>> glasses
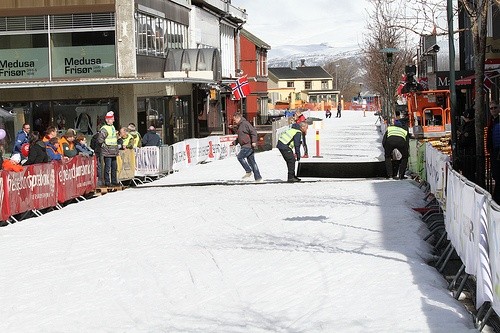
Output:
[489,106,498,109]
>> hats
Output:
[63,111,155,137]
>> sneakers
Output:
[392,174,407,180]
[243,172,262,182]
[288,176,301,182]
[105,182,120,187]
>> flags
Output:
[483,72,493,93]
[231,75,251,100]
[398,74,410,98]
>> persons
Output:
[325,109,332,118]
[292,110,310,159]
[336,101,342,117]
[230,112,263,183]
[455,108,475,183]
[91,125,104,184]
[96,110,122,188]
[488,99,500,206]
[284,108,290,117]
[382,120,410,181]
[0,123,95,170]
[276,121,309,182]
[394,111,402,127]
[115,122,160,150]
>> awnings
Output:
[453,71,491,85]
